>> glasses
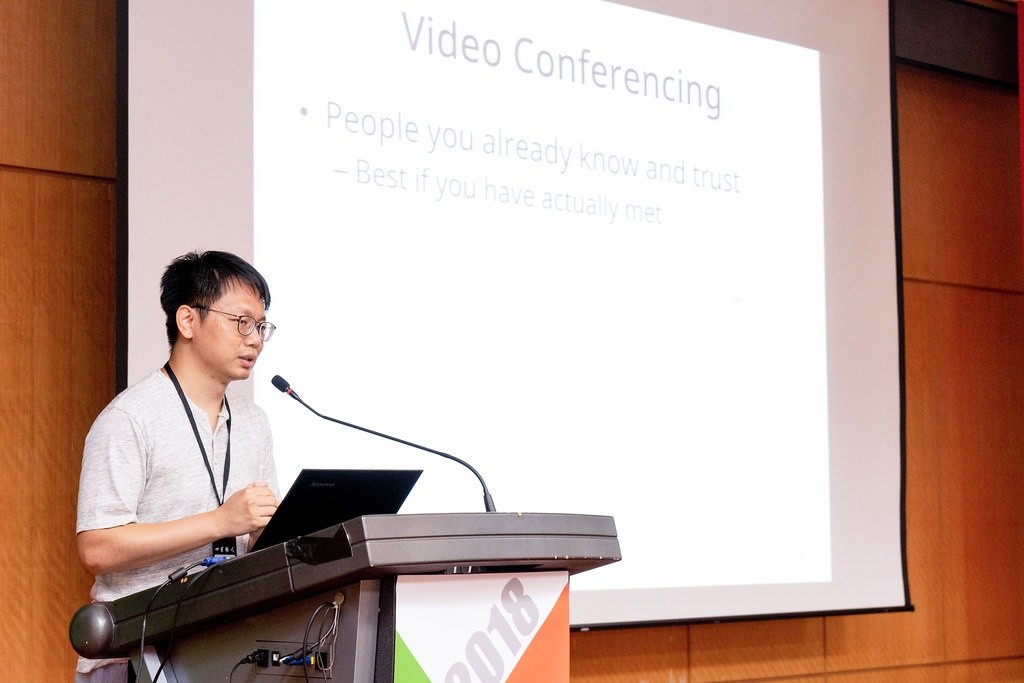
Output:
[192,305,276,342]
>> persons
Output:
[74,251,281,683]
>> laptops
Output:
[251,469,424,551]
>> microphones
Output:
[269,375,495,512]
